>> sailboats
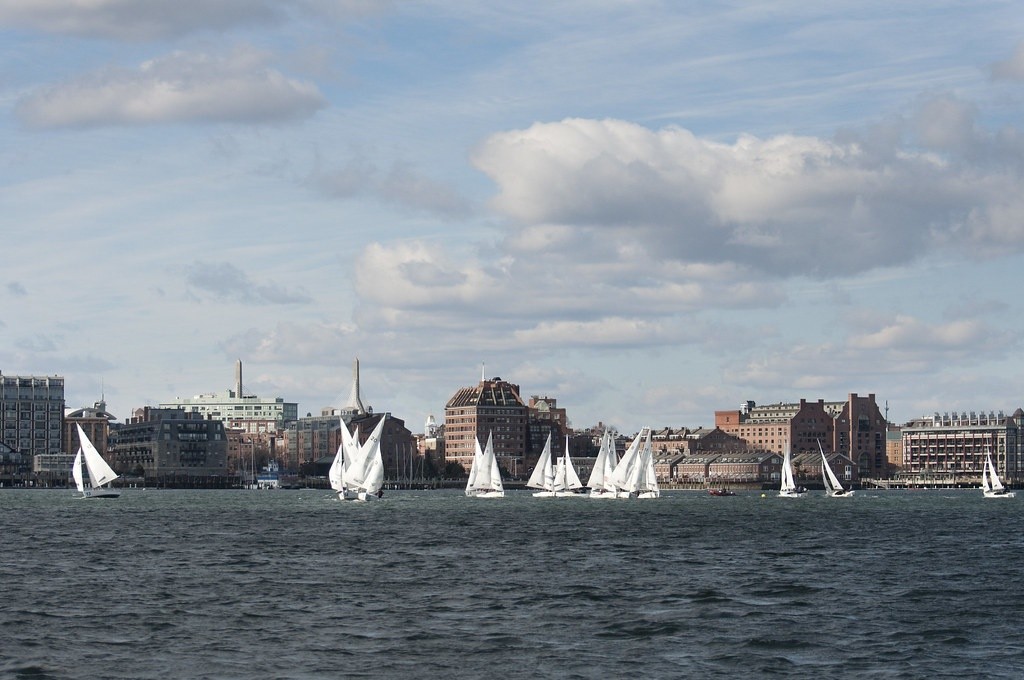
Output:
[816,438,855,498]
[464,428,505,497]
[585,427,663,499]
[525,429,592,499]
[72,421,122,498]
[328,411,386,501]
[776,442,808,498]
[982,437,1016,498]
